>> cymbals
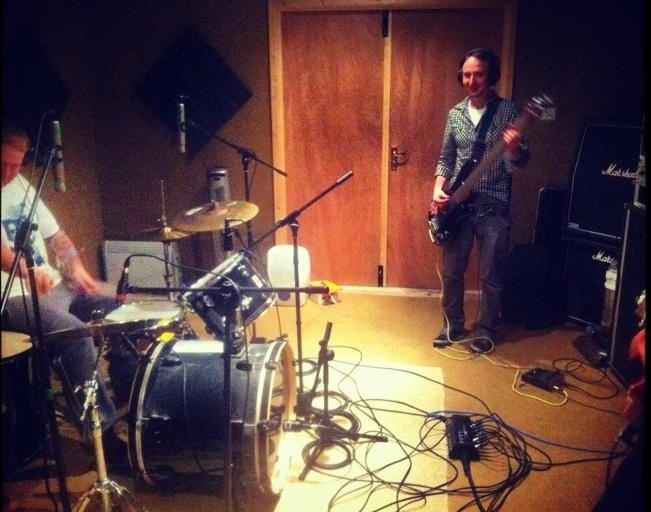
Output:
[23,316,162,343]
[170,200,259,232]
[148,226,197,240]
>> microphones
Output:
[179,102,187,155]
[116,258,130,302]
[224,220,233,258]
[50,120,66,193]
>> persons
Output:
[1,123,132,478]
[431,44,531,354]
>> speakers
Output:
[534,189,650,333]
[567,123,651,239]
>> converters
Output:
[521,372,561,392]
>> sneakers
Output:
[432,326,464,344]
[471,336,495,352]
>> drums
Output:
[126,337,297,496]
[105,301,199,361]
[183,252,278,340]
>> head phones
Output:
[457,49,500,86]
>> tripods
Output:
[71,333,148,512]
[298,320,388,480]
[248,171,361,428]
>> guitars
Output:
[427,92,555,247]
